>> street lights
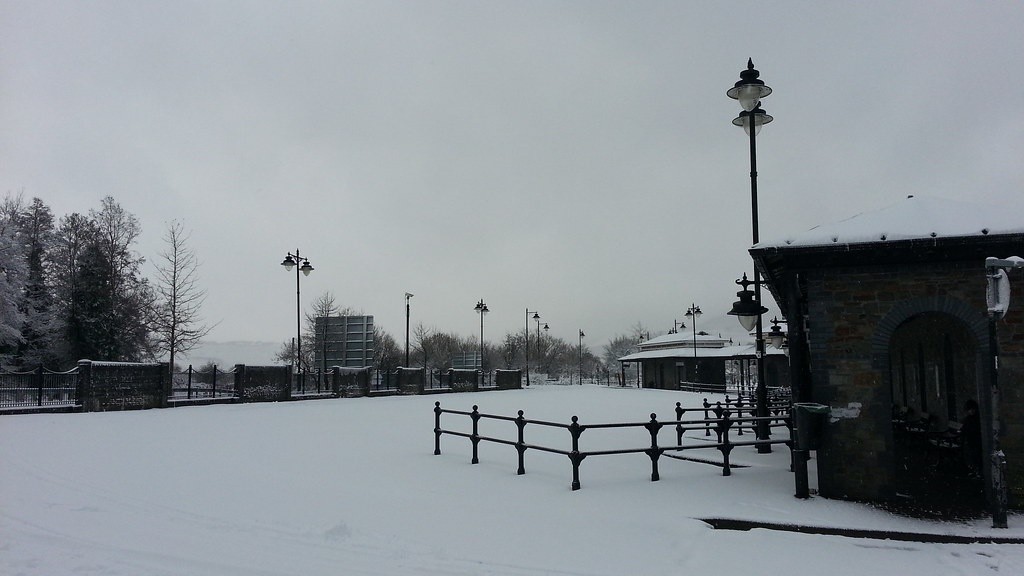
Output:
[473,298,491,387]
[537,321,550,353]
[282,249,315,391]
[674,319,686,334]
[640,331,650,342]
[684,302,704,384]
[525,307,541,387]
[726,55,776,455]
[578,329,586,386]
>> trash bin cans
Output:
[793,402,830,458]
